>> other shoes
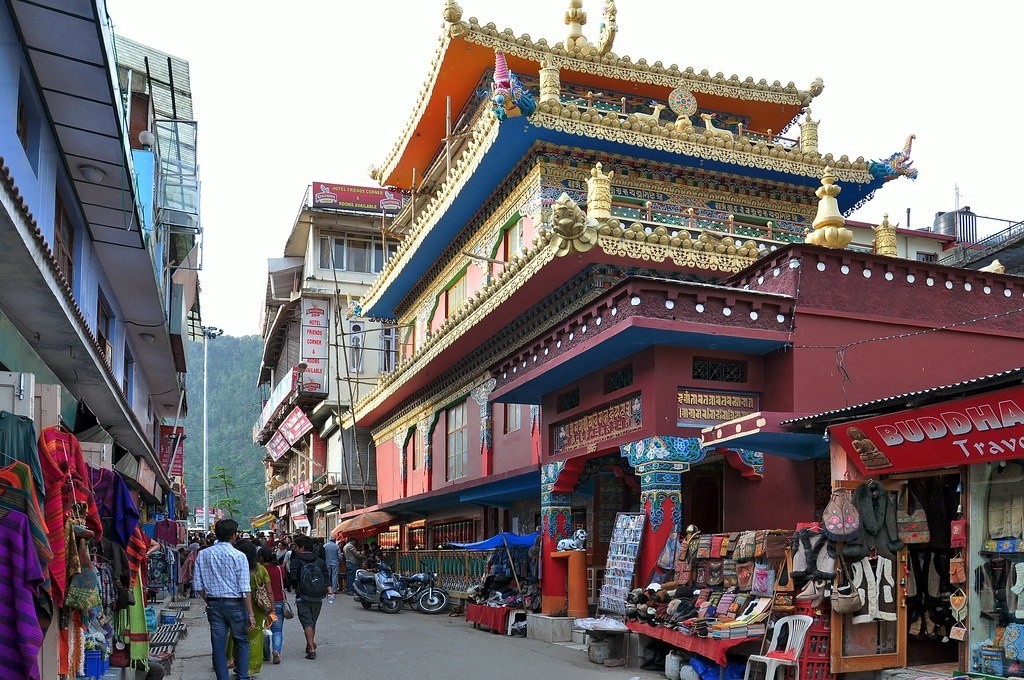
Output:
[306,642,316,653]
[273,651,281,664]
[263,656,271,661]
[306,652,316,659]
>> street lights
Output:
[198,326,225,535]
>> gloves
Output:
[655,603,668,619]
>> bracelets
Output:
[248,614,255,617]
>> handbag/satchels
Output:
[255,563,271,614]
[830,552,862,614]
[283,601,294,619]
[950,588,968,620]
[950,620,967,641]
[949,552,966,584]
[896,484,930,544]
[676,530,795,611]
[951,516,966,548]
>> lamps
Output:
[139,129,155,151]
[162,404,174,410]
[79,165,107,184]
[139,334,156,344]
[276,475,289,483]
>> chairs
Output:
[744,615,814,680]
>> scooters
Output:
[352,558,404,614]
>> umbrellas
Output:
[331,510,399,549]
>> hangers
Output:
[849,546,893,570]
[156,512,176,526]
[0,415,121,565]
[972,553,1019,576]
[149,539,177,554]
[797,522,831,536]
[852,479,892,492]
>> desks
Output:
[466,603,535,636]
[622,621,764,680]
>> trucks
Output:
[194,506,224,531]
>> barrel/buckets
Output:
[160,609,178,625]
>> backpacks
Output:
[298,559,325,600]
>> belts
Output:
[206,597,241,602]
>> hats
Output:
[674,586,695,600]
[242,533,251,538]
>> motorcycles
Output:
[391,560,450,615]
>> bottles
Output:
[327,596,334,603]
[973,658,979,672]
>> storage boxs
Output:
[784,600,837,680]
[75,641,110,680]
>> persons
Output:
[179,531,383,599]
[224,539,288,680]
[290,536,333,660]
[192,519,256,680]
